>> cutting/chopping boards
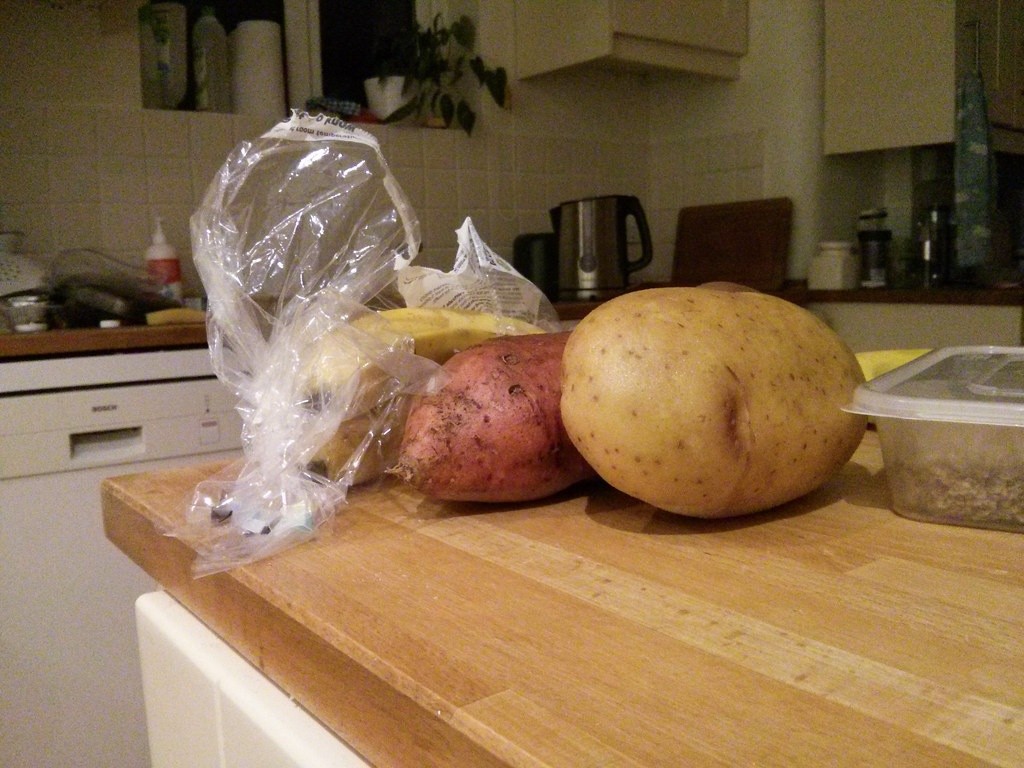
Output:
[670,196,792,298]
[99,428,1024,768]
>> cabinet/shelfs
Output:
[512,0,749,81]
[820,0,1024,156]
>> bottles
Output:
[192,6,231,111]
[805,240,860,291]
[144,219,184,307]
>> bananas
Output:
[296,306,552,488]
[849,347,935,382]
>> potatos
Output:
[560,285,869,519]
[390,332,619,504]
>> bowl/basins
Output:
[842,342,1024,535]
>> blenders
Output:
[856,206,892,289]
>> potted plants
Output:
[361,17,509,136]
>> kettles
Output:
[548,193,654,302]
